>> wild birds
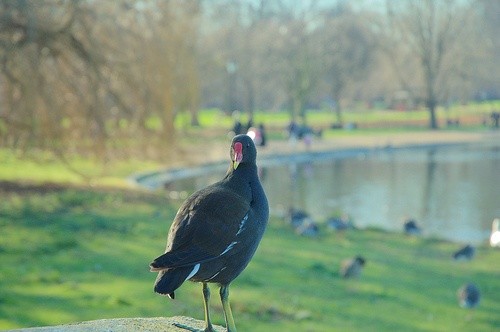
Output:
[458,284,479,306]
[403,221,420,235]
[489,218,500,248]
[287,208,308,224]
[149,134,269,332]
[452,245,475,261]
[338,256,365,278]
[295,223,319,237]
[325,216,347,232]
